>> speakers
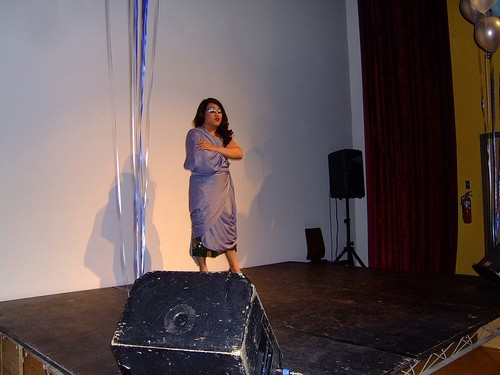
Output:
[110,270,284,375]
[472,241,500,284]
[328,148,365,200]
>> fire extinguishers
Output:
[460,190,474,224]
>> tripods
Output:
[335,199,367,268]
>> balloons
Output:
[459,0,500,53]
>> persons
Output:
[183,97,246,278]
